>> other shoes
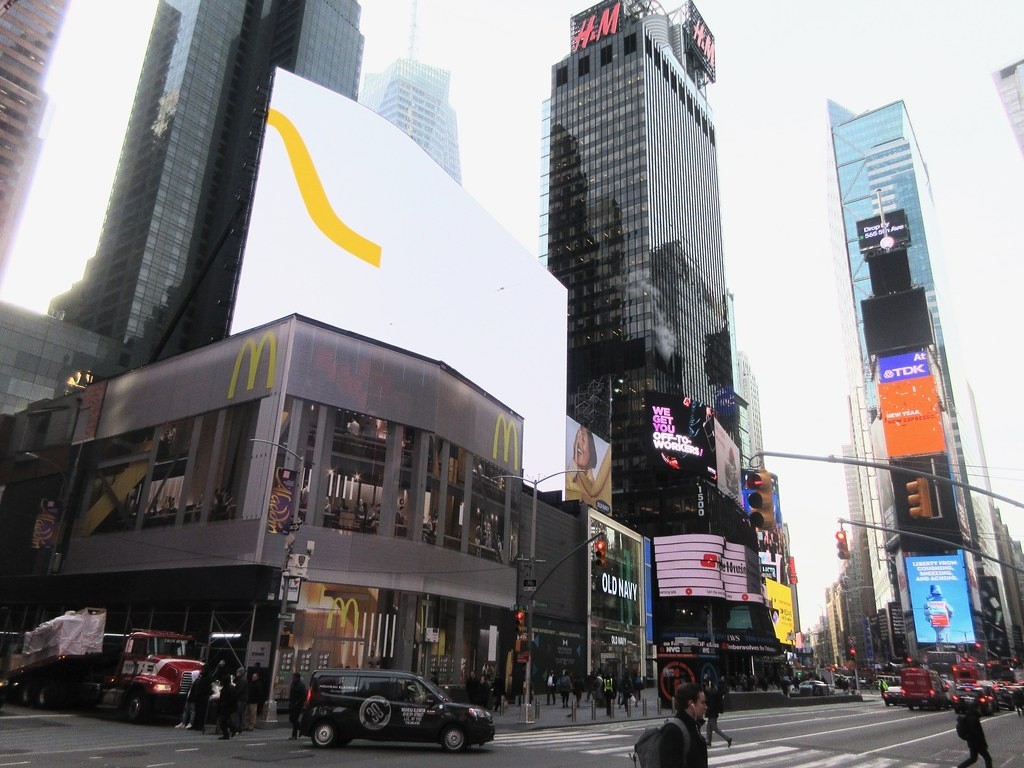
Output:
[175,722,186,729]
[219,736,232,740]
[727,738,732,747]
[186,724,192,729]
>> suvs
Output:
[302,667,497,753]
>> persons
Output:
[1012,687,1024,717]
[402,434,414,468]
[703,680,733,747]
[660,682,708,768]
[586,668,617,716]
[466,668,505,713]
[618,670,645,709]
[349,414,359,436]
[129,422,205,514]
[573,672,585,707]
[175,659,264,740]
[956,698,993,768]
[566,425,596,505]
[325,497,517,561]
[559,671,573,708]
[880,679,888,698]
[509,662,536,706]
[837,675,857,694]
[289,672,308,739]
[729,671,770,691]
[546,668,556,705]
[923,585,953,643]
[475,460,504,488]
[775,671,828,695]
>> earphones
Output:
[692,703,695,710]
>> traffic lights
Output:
[905,476,934,520]
[904,653,912,664]
[851,649,856,654]
[836,530,851,559]
[748,471,776,531]
[832,667,836,672]
[596,541,607,567]
[515,611,524,633]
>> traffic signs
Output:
[286,522,303,533]
[276,613,295,622]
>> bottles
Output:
[928,584,950,628]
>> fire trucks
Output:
[0,630,223,723]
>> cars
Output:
[861,663,1024,718]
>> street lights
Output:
[250,436,307,725]
[490,469,588,723]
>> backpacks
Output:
[632,717,690,768]
[955,715,968,739]
